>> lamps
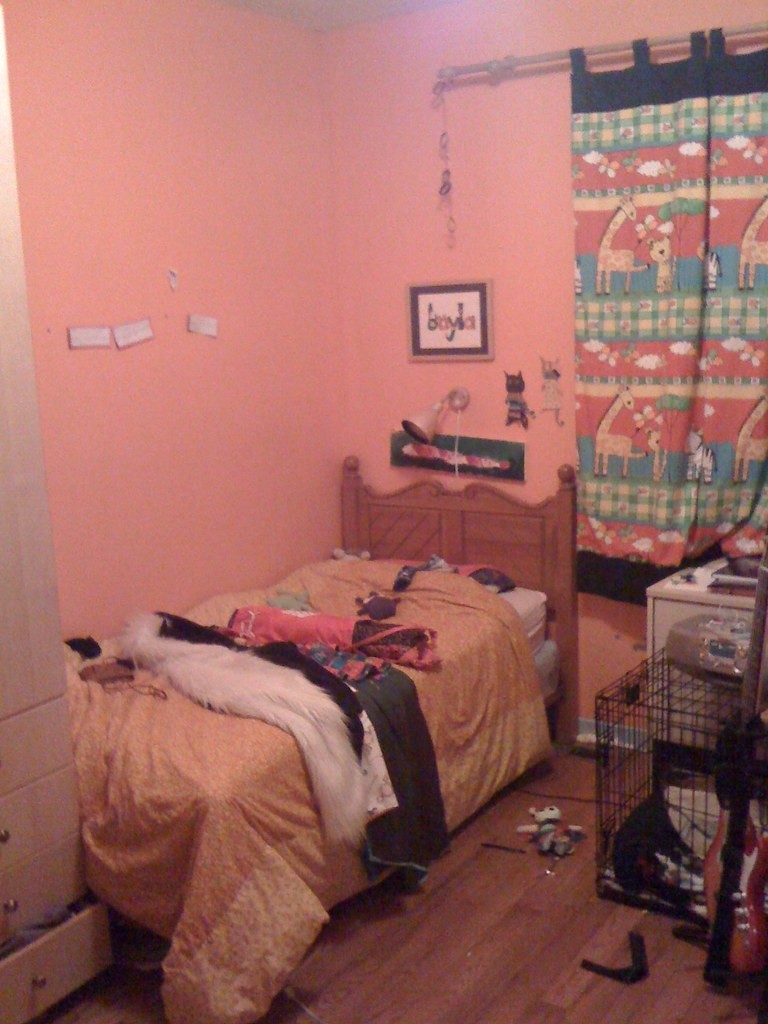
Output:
[404,384,471,444]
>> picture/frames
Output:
[409,280,497,363]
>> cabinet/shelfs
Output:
[643,564,766,755]
[1,0,115,1024]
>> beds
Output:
[61,452,579,1024]
